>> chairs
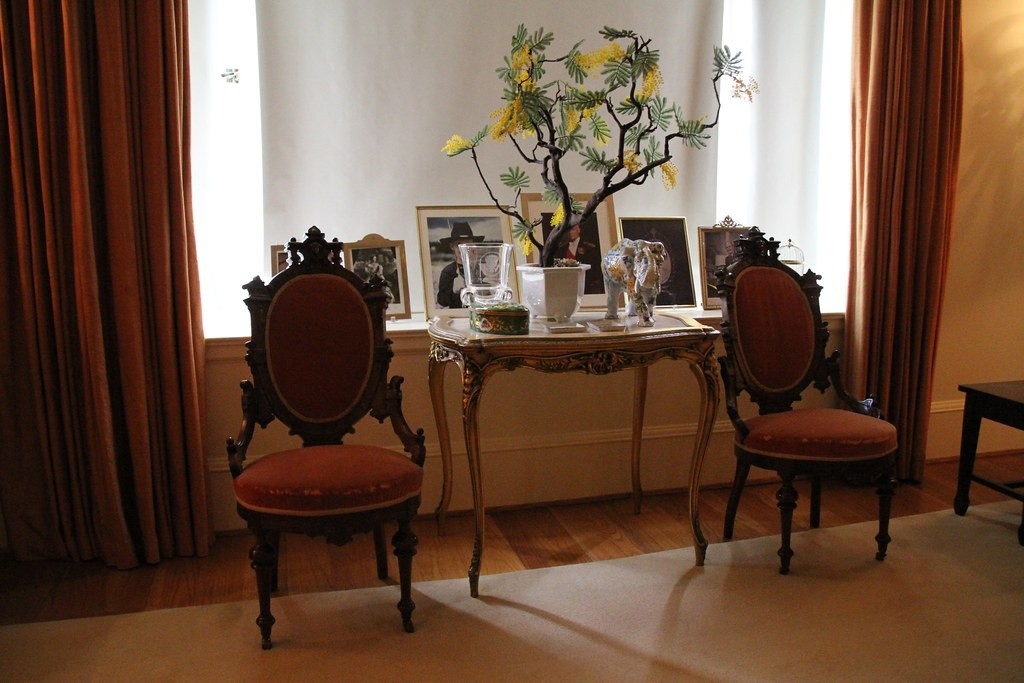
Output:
[714,225,901,573]
[226,226,429,649]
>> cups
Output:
[458,244,513,305]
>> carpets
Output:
[0,495,1024,683]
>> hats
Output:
[440,222,485,244]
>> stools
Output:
[951,376,1024,547]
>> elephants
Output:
[601,238,666,327]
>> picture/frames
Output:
[343,233,412,321]
[520,191,625,311]
[697,215,751,310]
[270,244,289,278]
[618,215,698,309]
[415,204,522,321]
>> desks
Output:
[425,307,722,596]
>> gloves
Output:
[453,278,465,294]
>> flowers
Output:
[438,15,761,265]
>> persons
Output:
[558,223,595,293]
[437,221,490,309]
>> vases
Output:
[514,258,593,318]
